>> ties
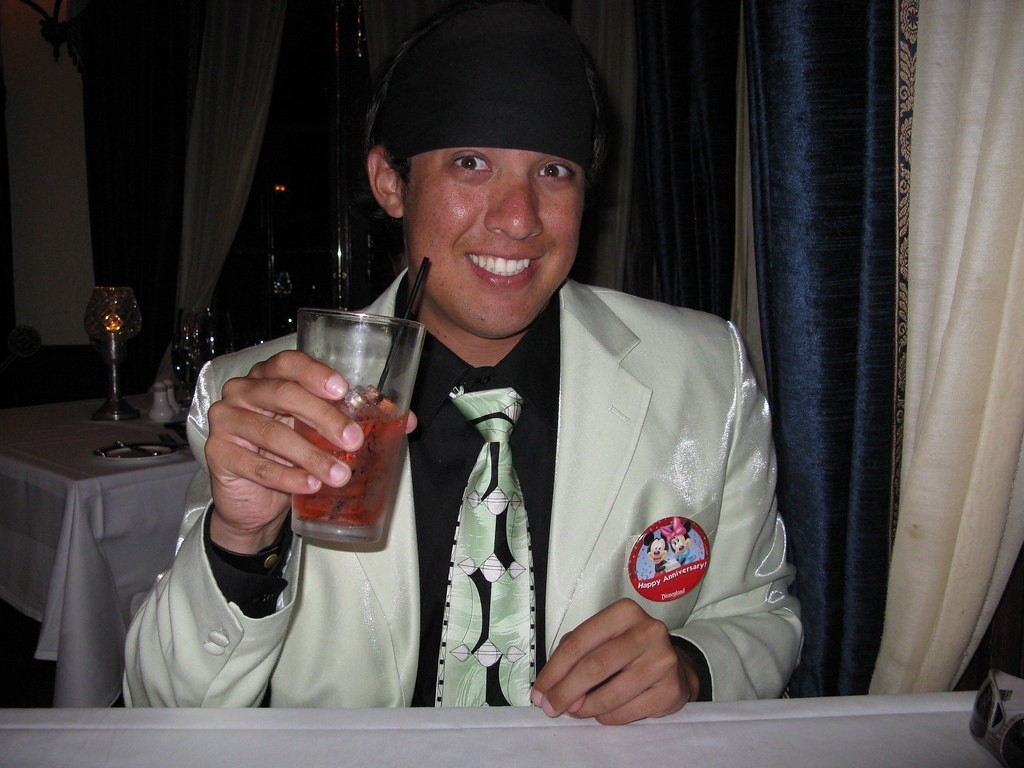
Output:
[432,385,536,706]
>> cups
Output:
[290,308,427,553]
[171,307,235,410]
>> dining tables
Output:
[0,394,201,708]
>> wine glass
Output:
[83,286,143,422]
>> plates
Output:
[93,439,184,460]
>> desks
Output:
[0,692,1023,768]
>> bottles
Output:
[148,377,180,423]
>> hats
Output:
[375,5,599,170]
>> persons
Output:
[120,1,805,726]
